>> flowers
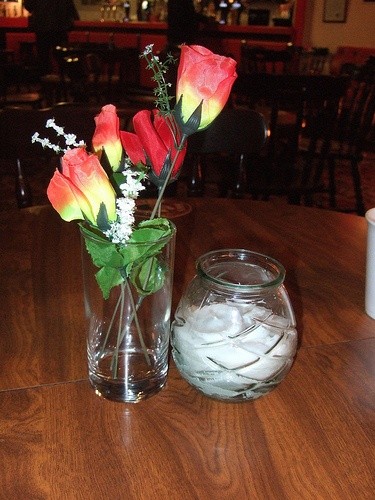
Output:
[32,44,237,379]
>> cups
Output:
[365,207,374,321]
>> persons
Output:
[165,0,221,110]
[193,0,217,22]
[22,0,80,79]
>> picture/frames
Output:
[322,0,348,23]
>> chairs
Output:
[186,45,375,217]
[0,42,139,174]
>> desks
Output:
[0,197,375,500]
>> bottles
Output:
[170,248,298,402]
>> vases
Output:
[78,215,177,405]
